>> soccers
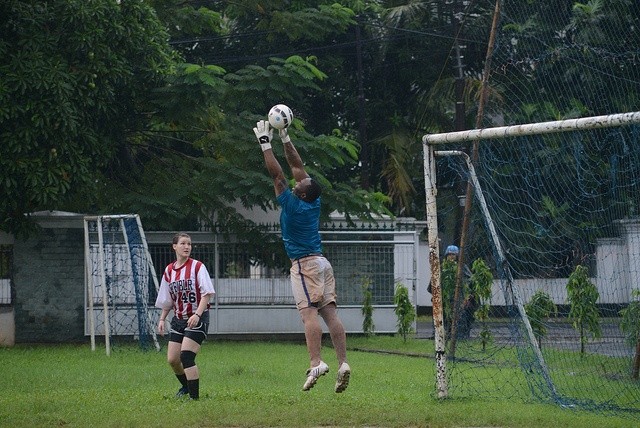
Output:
[268,103,294,130]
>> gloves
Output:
[252,119,274,153]
[279,126,291,145]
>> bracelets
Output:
[159,318,166,322]
[193,312,202,319]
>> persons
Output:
[154,231,217,403]
[252,119,352,394]
[427,244,482,342]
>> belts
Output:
[294,252,323,261]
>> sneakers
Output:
[334,362,351,394]
[303,361,329,391]
